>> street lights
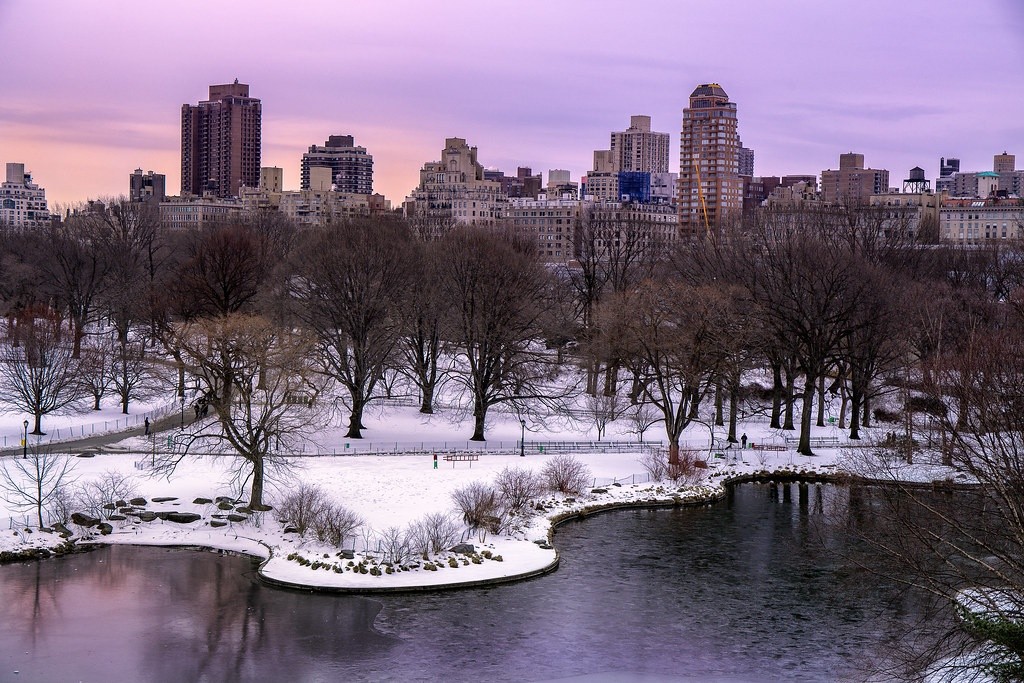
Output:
[23,420,30,458]
[711,413,715,445]
[275,415,281,450]
[520,420,526,456]
[928,415,933,448]
[179,398,185,431]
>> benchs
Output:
[787,437,838,445]
[522,440,664,450]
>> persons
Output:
[741,433,747,449]
[887,431,896,443]
[145,417,150,435]
[194,402,208,420]
